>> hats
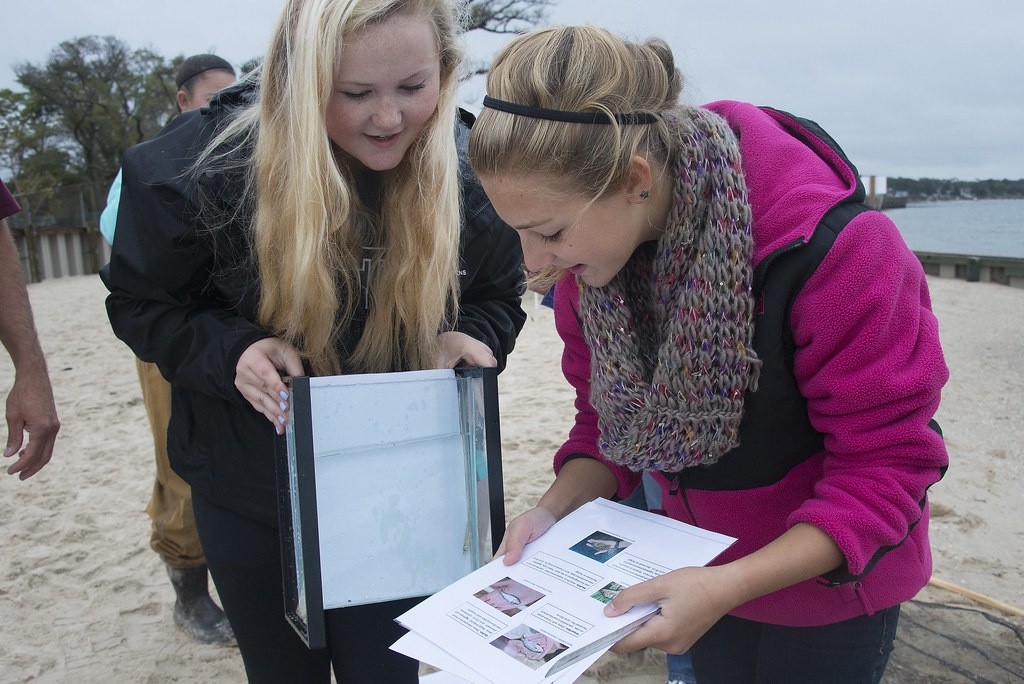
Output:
[177,55,235,89]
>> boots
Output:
[165,564,238,647]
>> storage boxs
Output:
[276,365,508,652]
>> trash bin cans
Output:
[966,257,981,281]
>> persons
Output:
[96,54,241,648]
[466,21,950,682]
[107,1,526,684]
[0,178,61,482]
